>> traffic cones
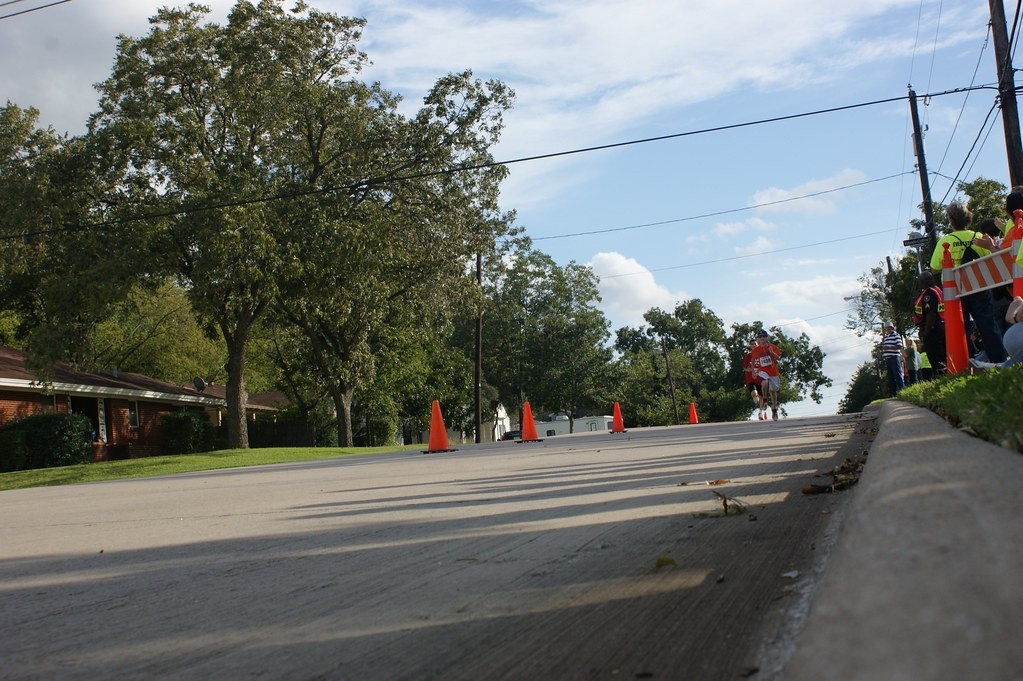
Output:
[609,402,628,434]
[687,403,701,424]
[515,400,544,443]
[421,400,460,454]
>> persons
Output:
[901,338,916,386]
[901,334,932,382]
[899,348,910,389]
[876,322,904,397]
[924,184,1023,377]
[744,338,766,419]
[750,330,781,420]
[914,272,946,380]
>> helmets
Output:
[918,271,933,288]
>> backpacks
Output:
[950,231,980,266]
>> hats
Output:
[883,322,894,330]
[756,330,768,337]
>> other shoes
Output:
[761,398,767,410]
[772,408,778,421]
[753,397,759,404]
[758,413,763,420]
